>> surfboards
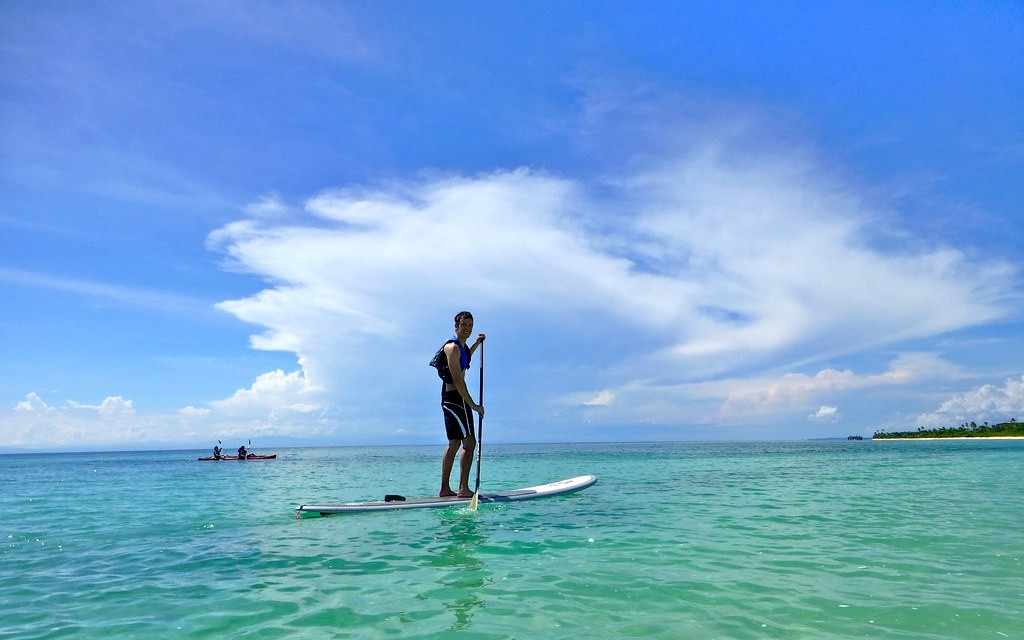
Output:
[294,474,599,512]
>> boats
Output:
[198,455,276,461]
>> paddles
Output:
[217,439,224,461]
[470,341,484,511]
[245,438,251,460]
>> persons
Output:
[214,446,222,457]
[440,311,486,497]
[238,446,247,458]
